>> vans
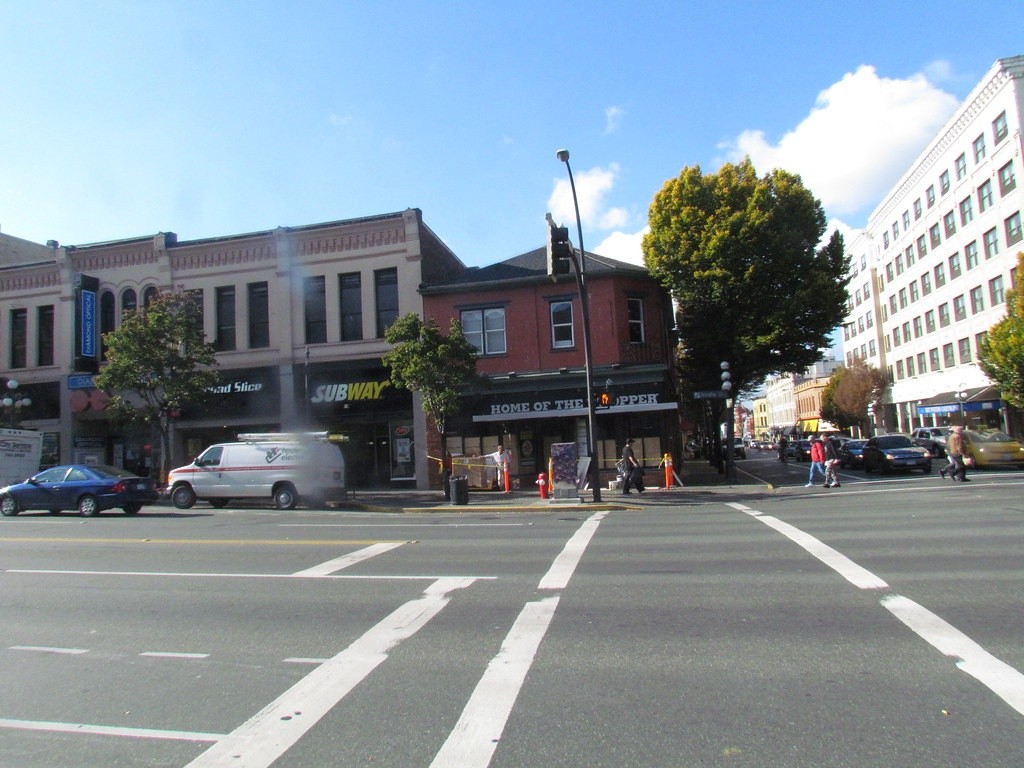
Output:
[167,432,348,511]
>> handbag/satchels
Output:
[961,454,972,466]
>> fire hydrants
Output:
[535,471,548,499]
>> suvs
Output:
[911,426,953,459]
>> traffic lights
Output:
[546,223,570,283]
[599,391,609,406]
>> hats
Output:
[626,439,636,443]
[806,435,814,442]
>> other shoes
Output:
[831,482,834,485]
[623,492,632,494]
[805,484,815,487]
[947,472,956,481]
[939,470,944,480]
[823,484,829,488]
[640,489,647,494]
[832,483,839,487]
[960,478,971,482]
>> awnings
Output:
[784,425,796,435]
[918,384,1003,414]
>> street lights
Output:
[0,378,33,430]
[954,391,968,430]
[556,145,602,502]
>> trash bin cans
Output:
[450,473,469,505]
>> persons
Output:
[778,437,788,460]
[949,426,972,482]
[936,429,955,479]
[820,433,841,488]
[804,435,825,487]
[622,439,646,494]
[481,446,511,490]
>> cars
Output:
[0,462,158,518]
[861,434,933,475]
[839,438,870,470]
[719,433,851,463]
[958,425,1024,470]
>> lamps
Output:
[970,363,976,366]
[611,364,620,369]
[911,376,918,379]
[345,400,349,410]
[559,368,569,374]
[938,370,943,374]
[508,372,518,378]
